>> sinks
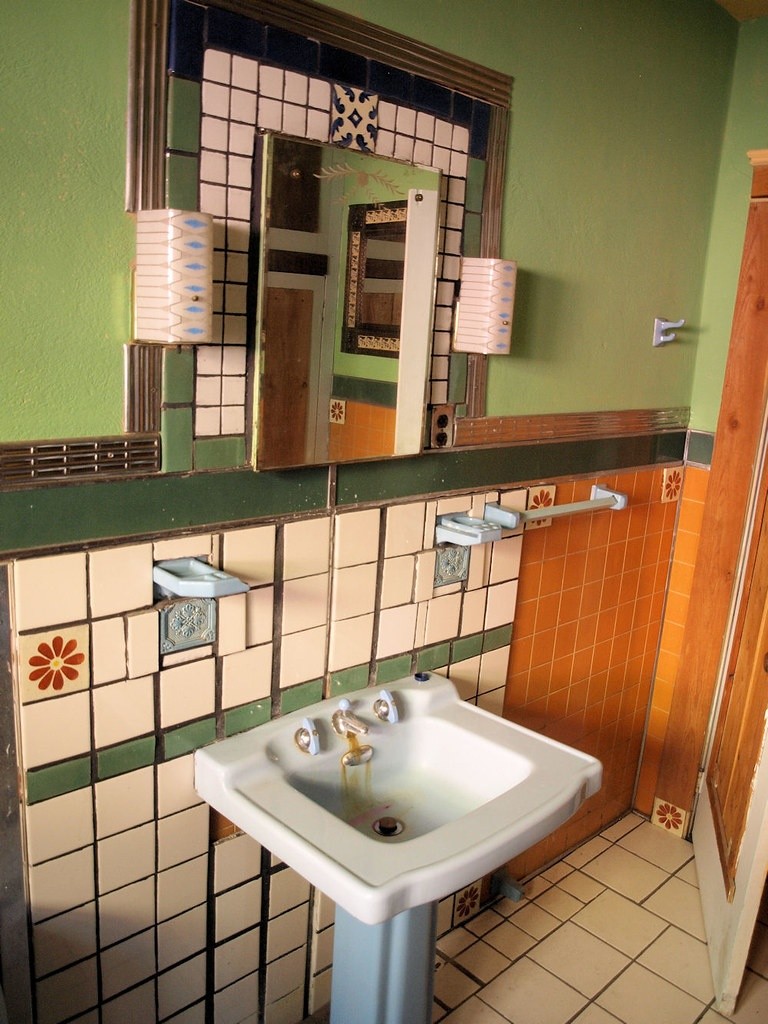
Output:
[193,669,603,926]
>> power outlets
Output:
[428,403,454,449]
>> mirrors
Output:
[122,0,516,436]
[340,199,408,359]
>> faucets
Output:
[331,697,370,736]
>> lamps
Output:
[134,208,213,355]
[452,256,517,356]
